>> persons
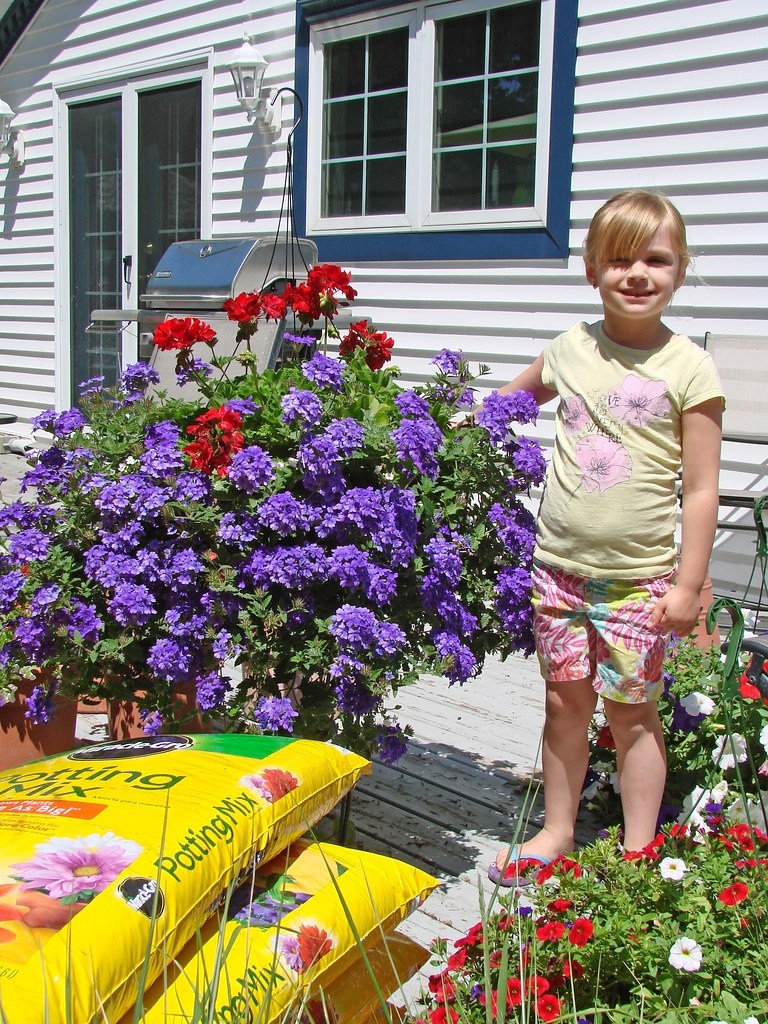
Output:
[472,189,726,887]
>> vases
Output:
[102,658,220,740]
[0,662,85,773]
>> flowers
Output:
[393,620,767,1023]
[0,263,550,764]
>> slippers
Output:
[489,843,555,887]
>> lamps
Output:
[225,27,283,135]
